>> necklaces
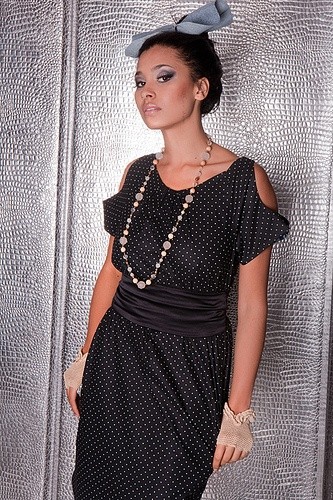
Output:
[118,132,214,289]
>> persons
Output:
[60,1,293,500]
